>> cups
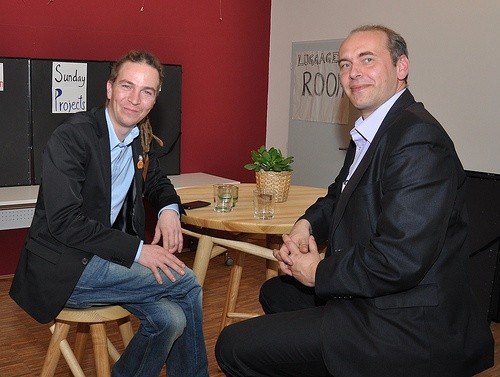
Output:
[252,189,275,220]
[213,184,239,214]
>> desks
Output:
[0,172,240,276]
[176,184,328,333]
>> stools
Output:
[40,306,133,377]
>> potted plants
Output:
[244,146,294,202]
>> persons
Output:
[214,22,496,377]
[8,50,208,377]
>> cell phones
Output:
[181,201,211,210]
